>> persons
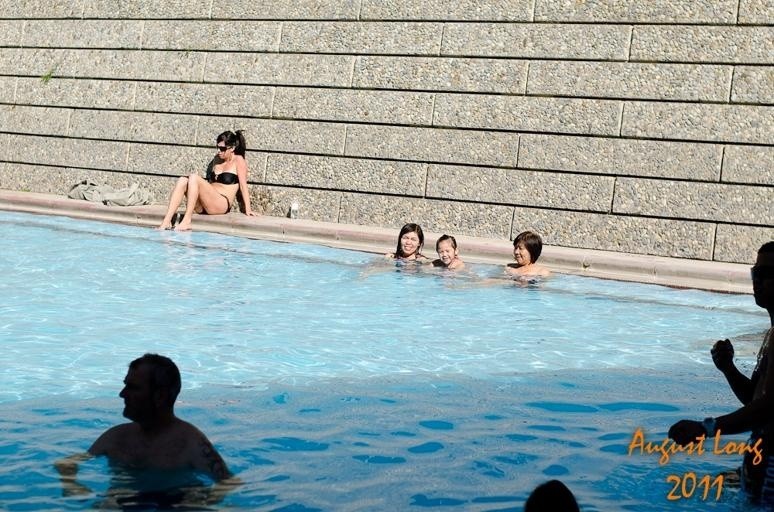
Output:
[154,128,261,233]
[51,353,247,505]
[668,240,773,510]
[383,222,430,264]
[499,230,551,276]
[429,234,465,272]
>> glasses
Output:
[751,264,774,280]
[217,145,231,151]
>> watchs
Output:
[702,417,716,436]
